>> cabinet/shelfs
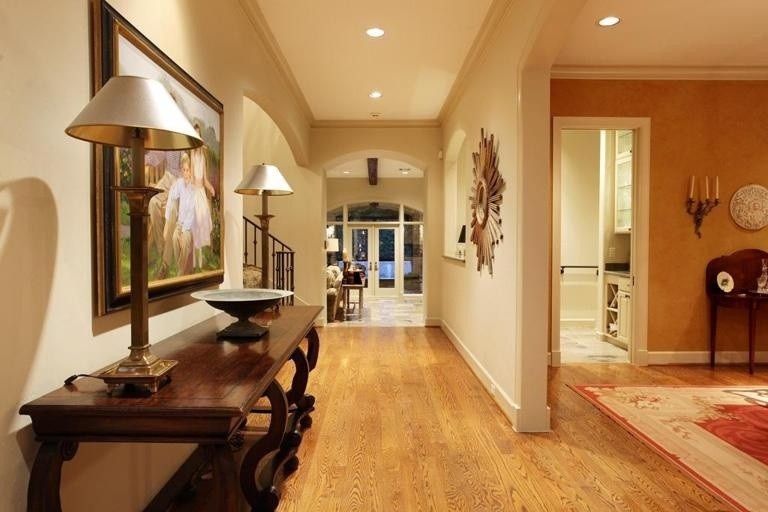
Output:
[603,273,631,351]
[614,129,632,234]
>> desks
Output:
[341,277,368,320]
[16,302,326,512]
[706,248,768,374]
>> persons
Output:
[148,123,215,279]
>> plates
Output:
[716,271,735,294]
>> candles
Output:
[689,176,695,199]
[705,175,710,199]
[715,175,719,199]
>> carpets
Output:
[327,295,425,328]
[565,383,768,512]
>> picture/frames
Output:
[86,0,225,318]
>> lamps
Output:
[234,162,295,312]
[67,74,205,401]
